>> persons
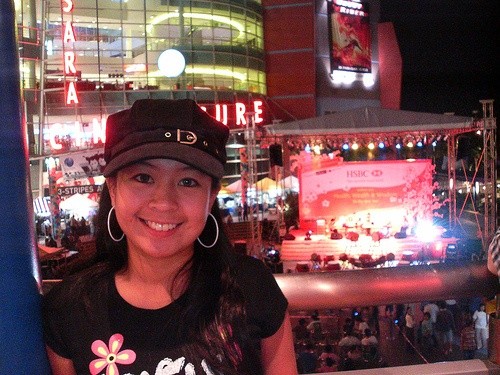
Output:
[227,201,249,225]
[286,212,420,274]
[291,300,490,375]
[44,100,298,375]
[35,209,97,278]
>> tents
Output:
[216,175,300,195]
[37,243,68,279]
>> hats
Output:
[103,99,229,180]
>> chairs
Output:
[294,331,384,369]
